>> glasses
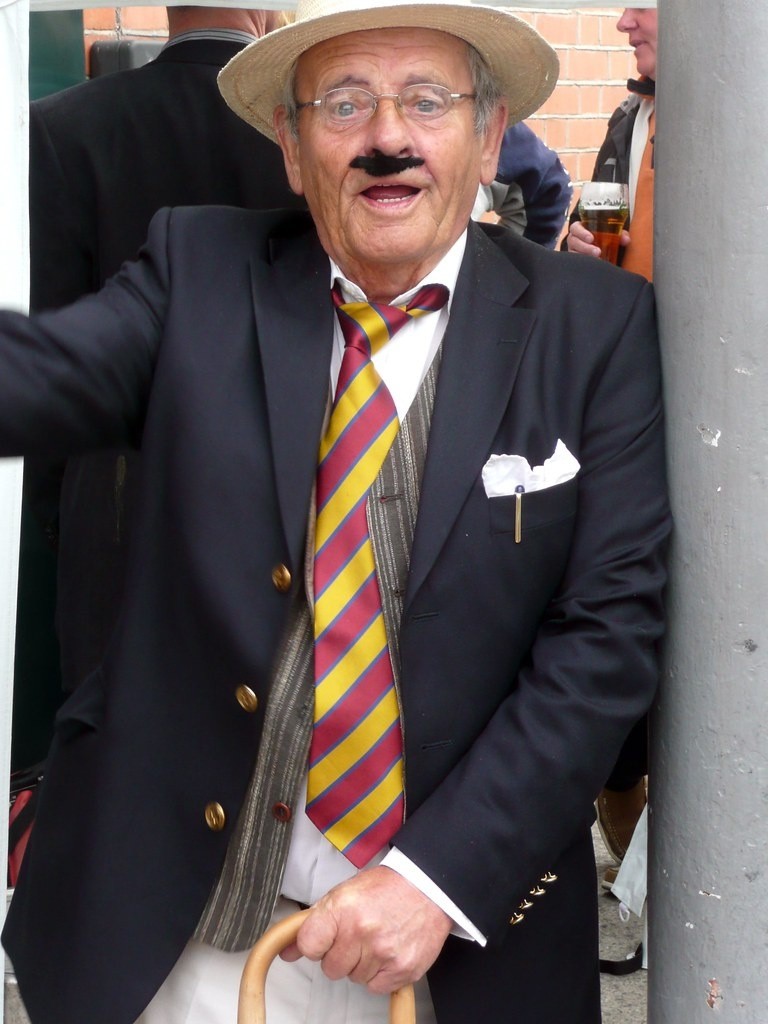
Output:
[294,83,481,125]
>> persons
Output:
[561,8,657,974]
[1,0,666,1023]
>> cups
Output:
[578,181,629,266]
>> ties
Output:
[304,278,449,871]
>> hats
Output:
[217,0,560,149]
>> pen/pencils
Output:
[514,484,526,543]
